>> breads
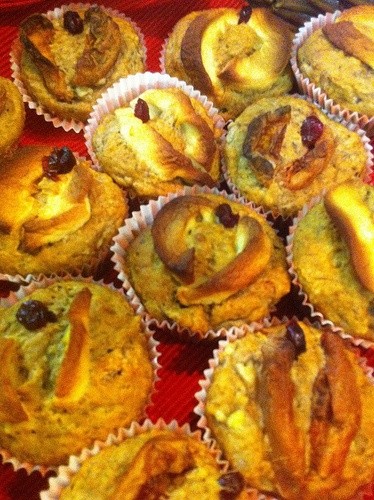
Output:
[0,5,373,500]
